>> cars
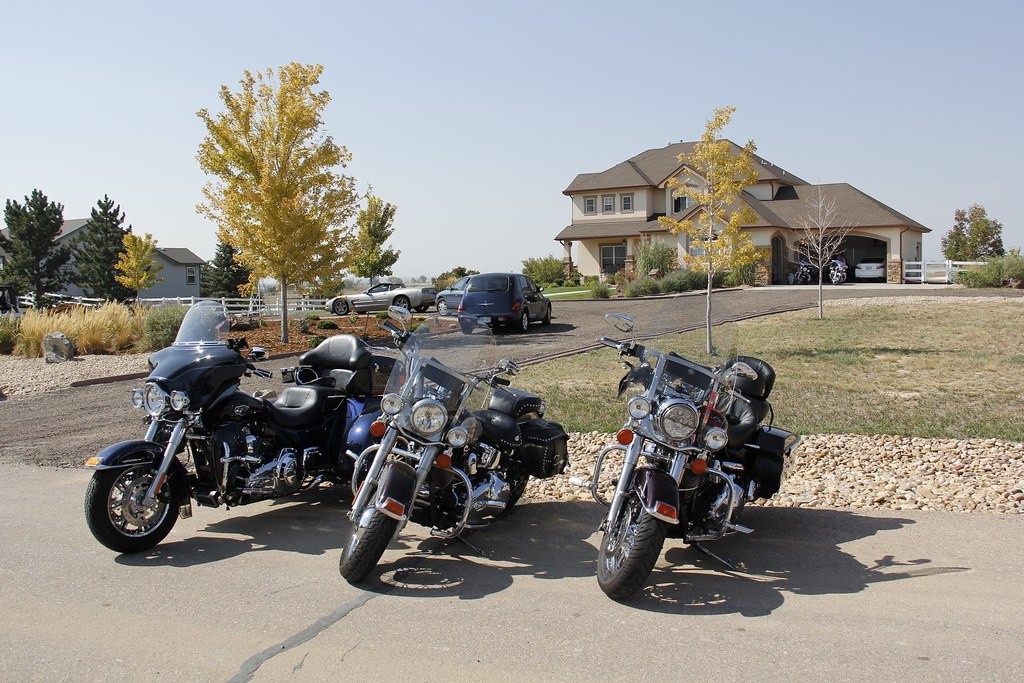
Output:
[458,275,552,334]
[435,276,475,316]
[855,257,886,282]
[325,283,436,316]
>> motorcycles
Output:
[591,314,804,602]
[829,257,849,284]
[339,305,570,583]
[794,257,814,284]
[84,278,407,554]
